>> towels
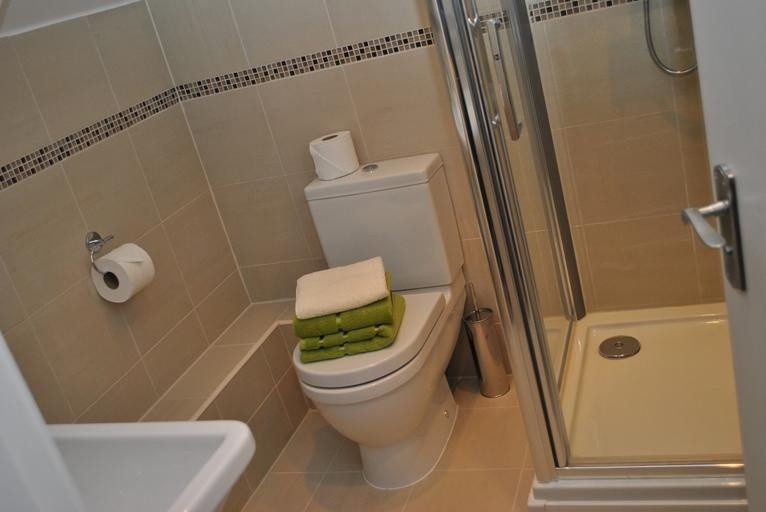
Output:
[292,254,407,364]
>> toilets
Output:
[292,151,468,488]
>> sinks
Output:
[45,419,258,511]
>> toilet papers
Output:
[309,129,360,182]
[90,242,156,304]
